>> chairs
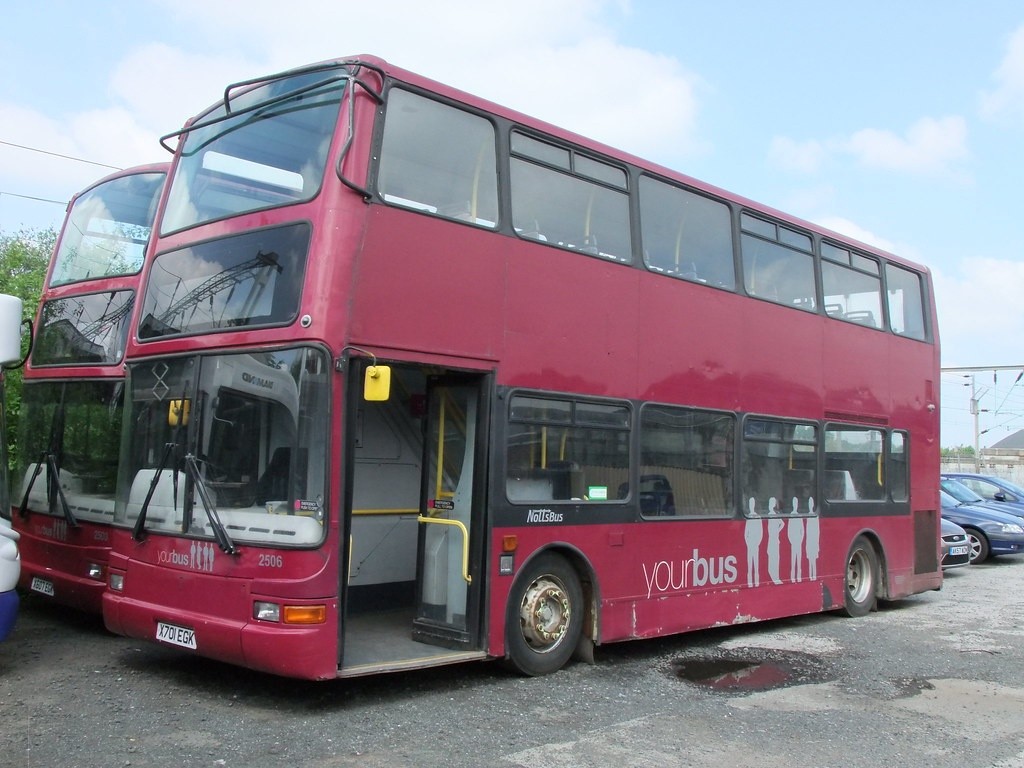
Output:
[255,446,308,507]
[378,179,876,329]
[518,459,904,516]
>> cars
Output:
[940,472,1024,571]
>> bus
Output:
[99,54,941,702]
[10,156,184,636]
